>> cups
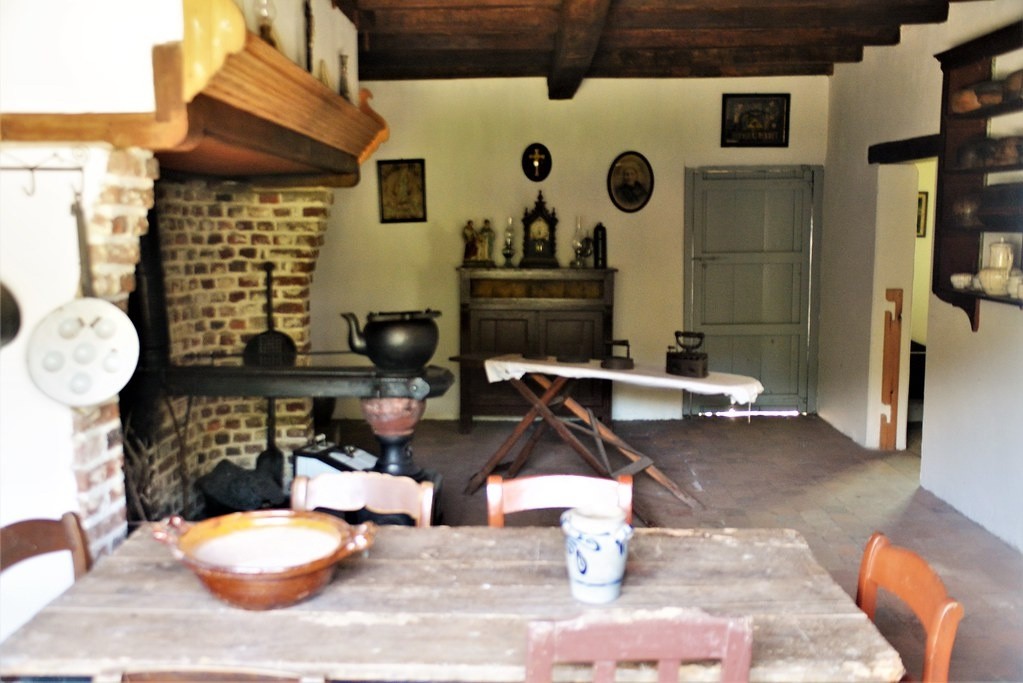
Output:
[559,505,635,604]
[949,236,1023,300]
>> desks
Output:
[0,520,906,683]
[151,357,454,404]
[446,352,766,528]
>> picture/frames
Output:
[917,191,928,238]
[720,90,791,149]
[607,150,655,213]
[376,156,429,224]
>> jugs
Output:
[339,309,441,373]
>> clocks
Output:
[518,189,561,269]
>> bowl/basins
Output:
[153,507,374,611]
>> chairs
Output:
[290,468,437,530]
[480,469,639,526]
[855,531,968,683]
[520,602,760,683]
[0,508,94,589]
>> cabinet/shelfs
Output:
[927,18,1023,336]
[454,263,621,439]
